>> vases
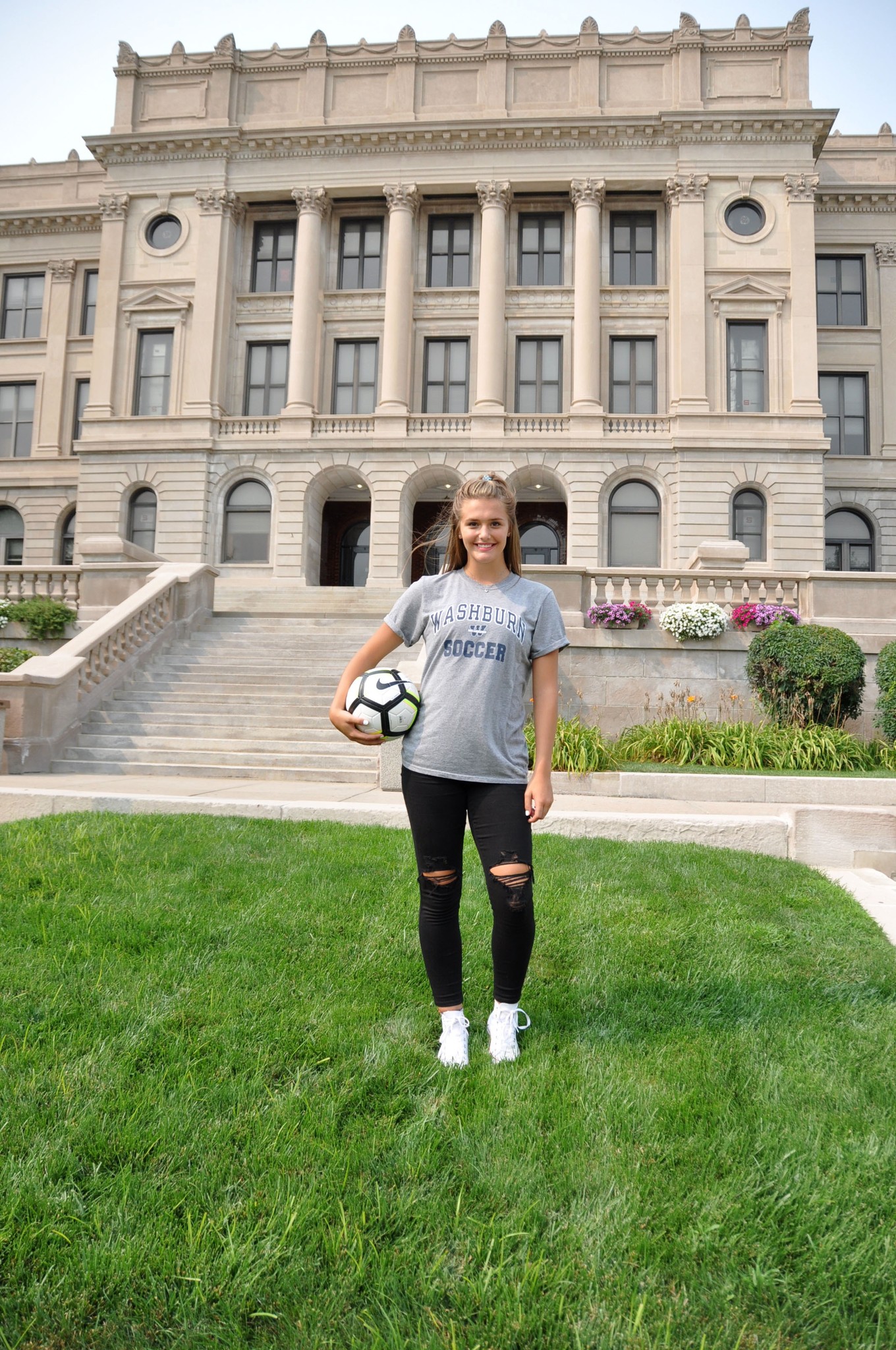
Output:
[731,620,767,633]
[596,618,640,630]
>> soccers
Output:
[345,666,422,744]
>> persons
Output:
[329,472,572,1067]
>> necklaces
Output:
[464,565,507,593]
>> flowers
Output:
[587,601,652,629]
[730,603,802,630]
[659,602,733,644]
[0,596,80,643]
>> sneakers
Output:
[486,999,531,1064]
[436,1008,470,1069]
